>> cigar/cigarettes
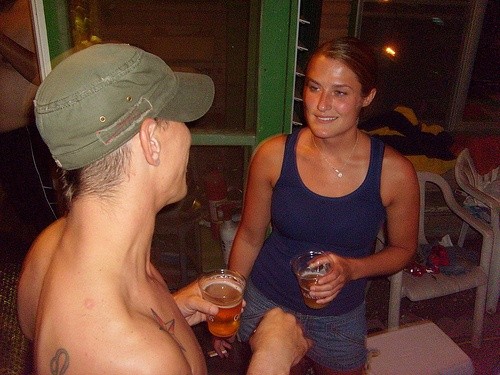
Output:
[210,349,227,358]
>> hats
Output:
[32,42,216,171]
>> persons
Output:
[213,35,421,375]
[18,43,314,375]
[0,0,57,268]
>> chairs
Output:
[366,171,495,350]
[456,137,500,314]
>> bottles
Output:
[219,205,238,264]
[207,171,232,240]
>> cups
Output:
[197,268,247,338]
[292,252,335,309]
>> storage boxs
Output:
[196,216,225,273]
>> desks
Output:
[364,327,475,375]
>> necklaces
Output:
[312,127,359,177]
